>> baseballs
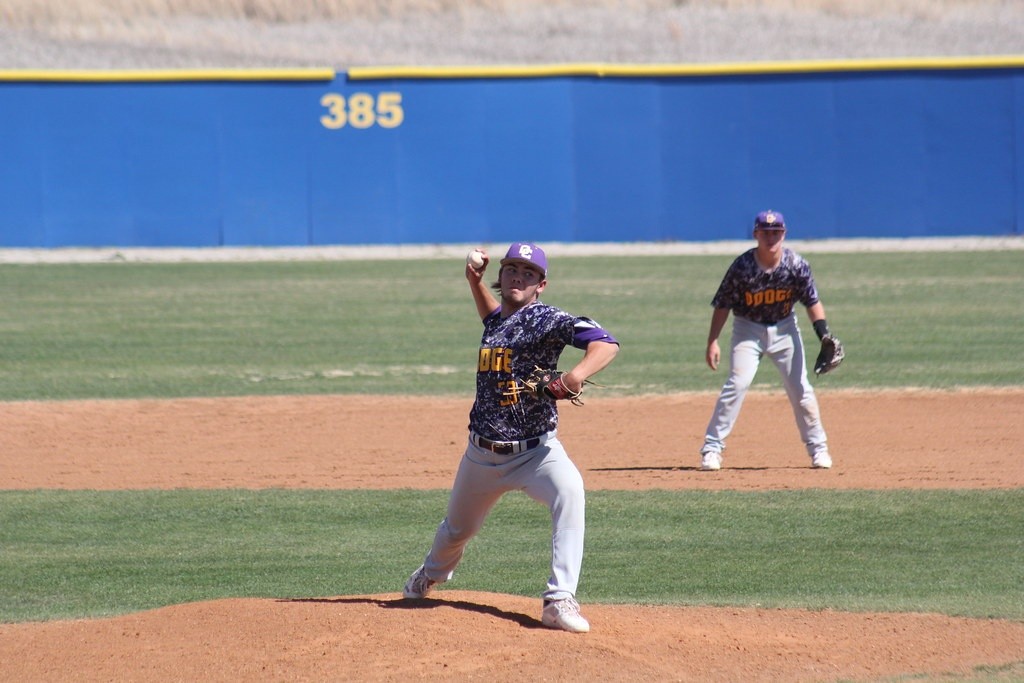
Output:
[466,249,485,269]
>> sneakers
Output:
[810,450,833,470]
[402,564,436,599]
[700,451,723,472]
[540,597,590,633]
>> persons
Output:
[697,208,847,472]
[401,239,622,634]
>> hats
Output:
[499,242,548,278]
[753,211,786,231]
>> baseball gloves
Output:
[813,333,846,375]
[523,368,584,402]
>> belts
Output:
[465,424,540,455]
